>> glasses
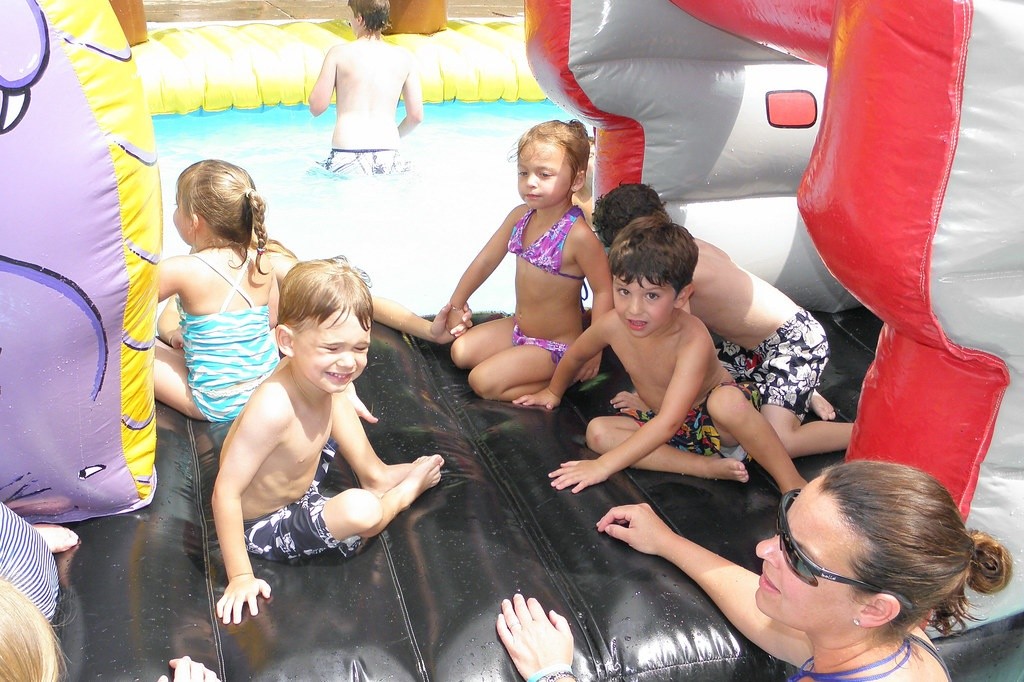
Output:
[776,488,915,611]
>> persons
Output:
[153,159,473,423]
[445,120,855,495]
[496,461,1013,682]
[308,0,423,175]
[1,500,222,682]
[211,258,445,625]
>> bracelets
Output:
[527,665,579,682]
[168,334,172,345]
[451,305,464,311]
